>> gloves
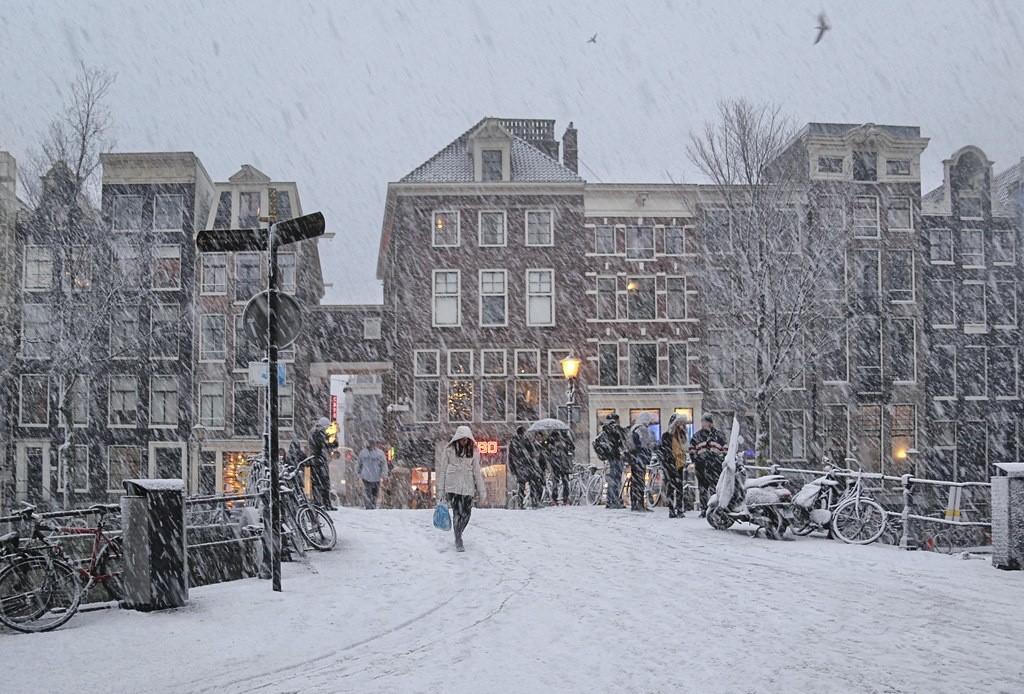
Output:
[480,491,487,500]
[436,491,446,500]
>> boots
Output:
[454,522,465,552]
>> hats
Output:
[319,418,332,427]
[701,412,714,423]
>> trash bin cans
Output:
[990,463,1024,571]
[118,479,188,613]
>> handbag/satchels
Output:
[433,499,451,531]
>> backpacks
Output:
[625,423,643,453]
[592,424,619,461]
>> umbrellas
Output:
[528,419,568,431]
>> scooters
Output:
[707,451,793,538]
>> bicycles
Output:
[0,501,123,633]
[883,510,991,554]
[786,457,887,545]
[257,454,336,557]
[508,458,667,509]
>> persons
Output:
[662,413,687,518]
[630,411,657,512]
[509,426,577,509]
[308,417,338,511]
[289,441,308,467]
[280,448,287,461]
[392,460,411,509]
[355,440,388,510]
[689,413,729,518]
[603,414,626,508]
[439,426,486,551]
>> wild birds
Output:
[813,14,831,44]
[588,34,597,44]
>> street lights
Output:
[560,354,582,431]
[191,423,205,499]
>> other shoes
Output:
[328,506,338,512]
[698,513,707,519]
